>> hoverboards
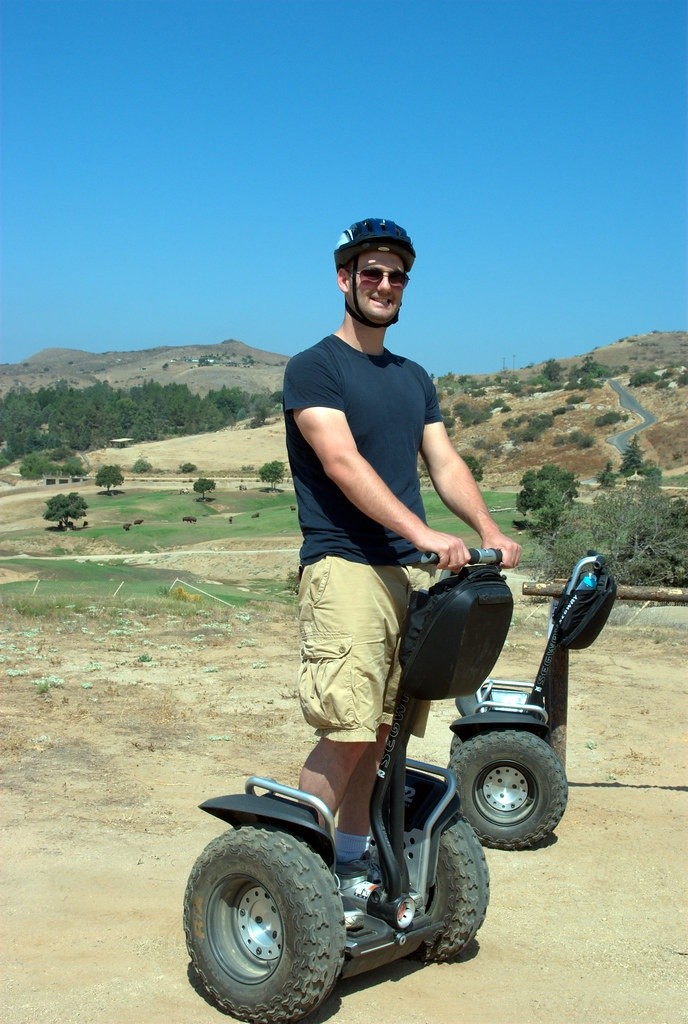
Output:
[444,549,619,851]
[182,547,514,1024]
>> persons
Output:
[283,218,522,931]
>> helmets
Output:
[334,217,417,273]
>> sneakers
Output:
[332,852,424,912]
[331,872,365,932]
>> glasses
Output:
[350,268,411,291]
